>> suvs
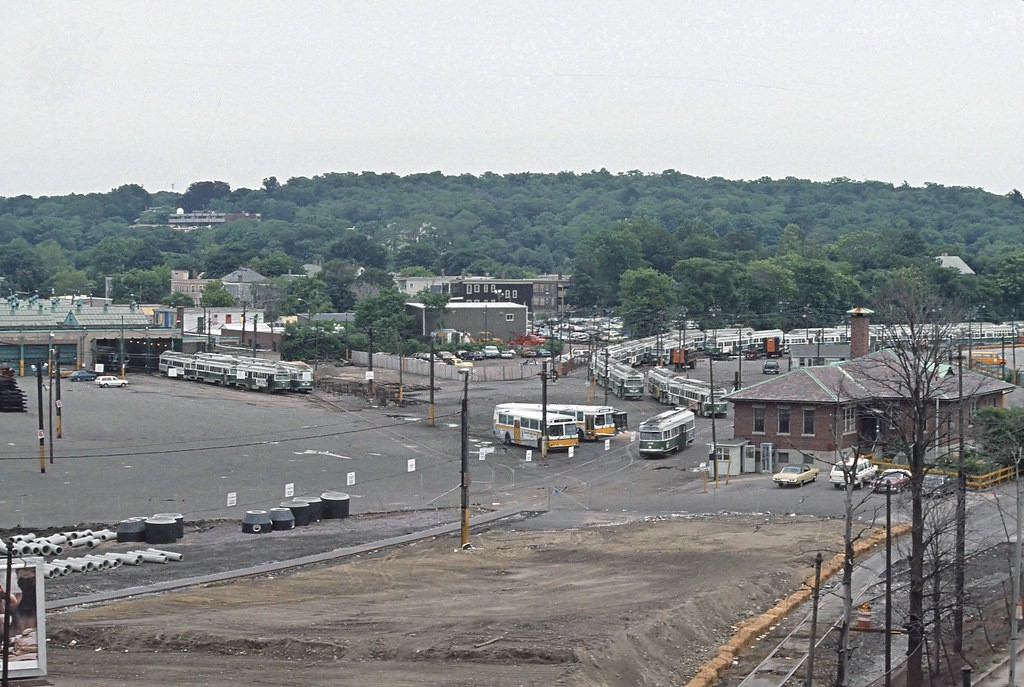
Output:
[95,376,129,388]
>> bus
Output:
[493,403,617,442]
[495,409,580,454]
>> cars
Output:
[67,371,97,382]
[410,345,551,367]
[52,367,75,378]
[869,469,912,494]
[531,317,628,343]
[920,474,957,498]
[772,464,820,488]
[763,361,779,374]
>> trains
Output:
[638,407,696,459]
[159,350,315,393]
[591,322,1024,419]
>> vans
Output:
[829,457,879,490]
[971,352,1006,368]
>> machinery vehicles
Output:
[463,330,504,344]
